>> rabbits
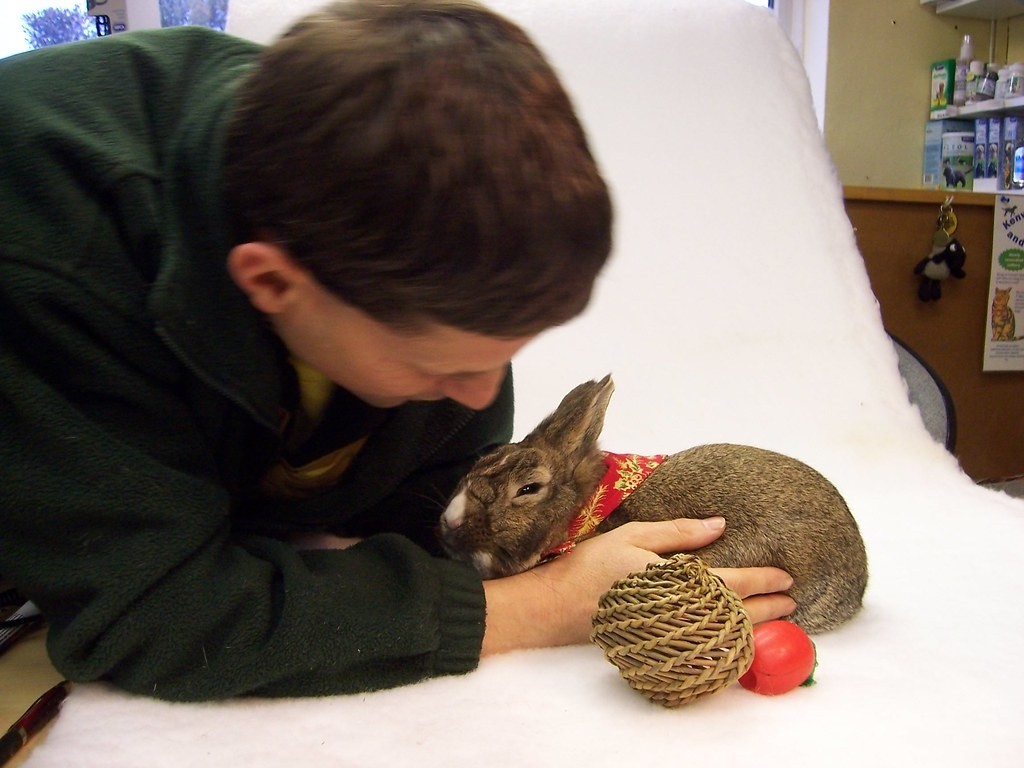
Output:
[436,374,869,636]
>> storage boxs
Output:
[923,59,1024,190]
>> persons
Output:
[1,0,798,704]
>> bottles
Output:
[977,64,999,99]
[1006,65,1024,97]
[954,35,977,105]
[994,70,1008,98]
[964,61,984,105]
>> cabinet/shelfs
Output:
[919,0,1024,120]
